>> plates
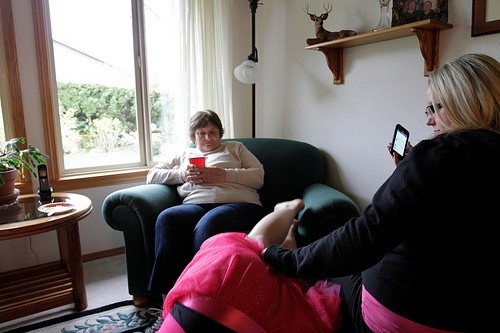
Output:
[38,202,74,214]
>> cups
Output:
[189,157,205,170]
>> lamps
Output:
[234,0,264,139]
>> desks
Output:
[0,193,94,325]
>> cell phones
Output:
[390,124,409,161]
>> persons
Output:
[146,109,264,333]
[154,199,341,333]
[400,0,435,25]
[261,54,500,333]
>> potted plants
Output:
[0,137,50,207]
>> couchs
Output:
[102,137,362,309]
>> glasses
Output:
[425,102,444,116]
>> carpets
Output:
[2,300,158,333]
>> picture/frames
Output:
[391,0,448,28]
